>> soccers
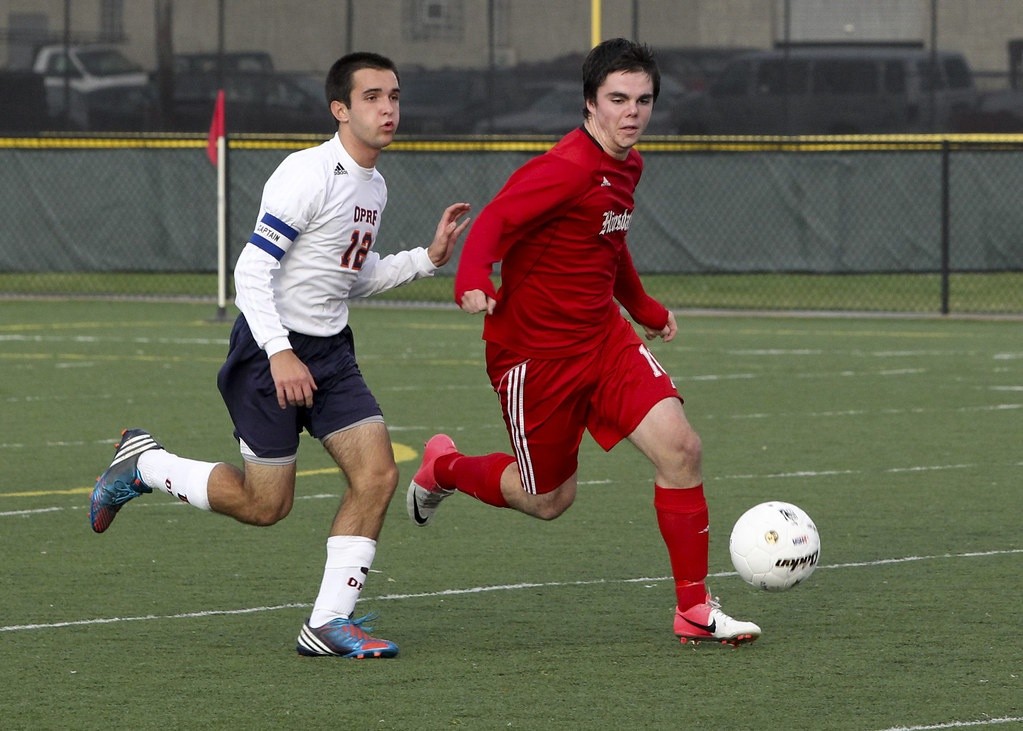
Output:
[730,501,821,593]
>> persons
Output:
[405,40,762,646]
[86,52,471,658]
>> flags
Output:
[206,90,226,164]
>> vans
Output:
[672,46,972,136]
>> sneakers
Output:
[674,587,761,648]
[406,434,457,527]
[86,429,164,534]
[295,612,398,659]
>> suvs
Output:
[151,49,312,131]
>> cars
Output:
[474,76,687,138]
[18,46,152,131]
